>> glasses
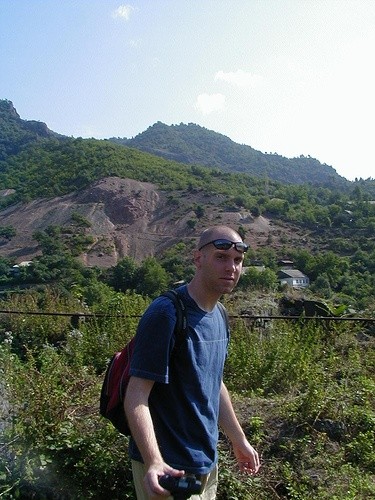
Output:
[199,237,250,252]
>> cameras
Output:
[158,471,201,500]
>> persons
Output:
[122,226,260,500]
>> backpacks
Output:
[100,290,187,438]
